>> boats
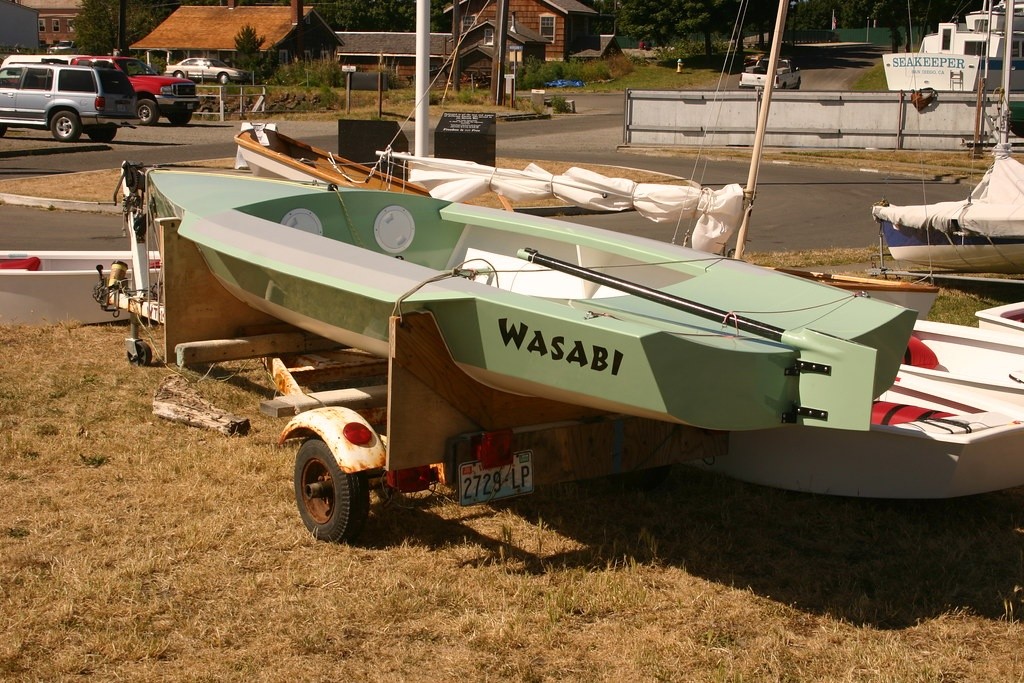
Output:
[144,162,921,437]
[234,120,430,204]
[0,246,164,329]
[678,296,1024,508]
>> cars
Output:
[164,57,251,85]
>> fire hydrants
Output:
[676,58,685,74]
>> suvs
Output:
[0,62,143,144]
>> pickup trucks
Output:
[739,57,802,90]
[1,54,202,127]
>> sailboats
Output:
[871,1,1024,271]
